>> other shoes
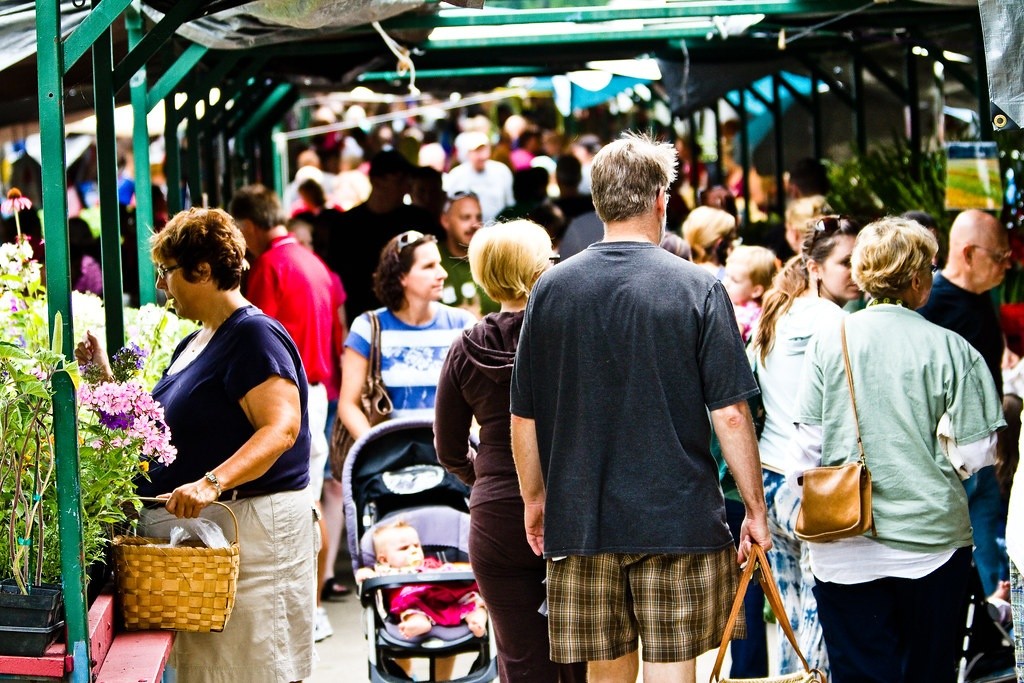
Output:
[315,606,333,641]
[321,576,353,602]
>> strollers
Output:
[342,414,498,683]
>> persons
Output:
[783,215,1010,683]
[510,128,774,683]
[73,207,316,683]
[224,77,1024,683]
[433,218,588,683]
[0,121,169,296]
[354,521,489,638]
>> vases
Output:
[0,585,60,657]
[2,576,64,637]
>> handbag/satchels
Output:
[794,461,872,543]
[329,311,393,484]
[709,544,827,683]
[749,371,766,441]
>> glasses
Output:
[550,254,560,264]
[157,264,182,279]
[809,213,851,255]
[917,263,938,275]
[974,244,1013,264]
[396,230,424,253]
[443,190,478,213]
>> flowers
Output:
[0,191,199,597]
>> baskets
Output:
[111,496,240,634]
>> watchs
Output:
[204,471,222,498]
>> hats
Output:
[463,131,489,151]
